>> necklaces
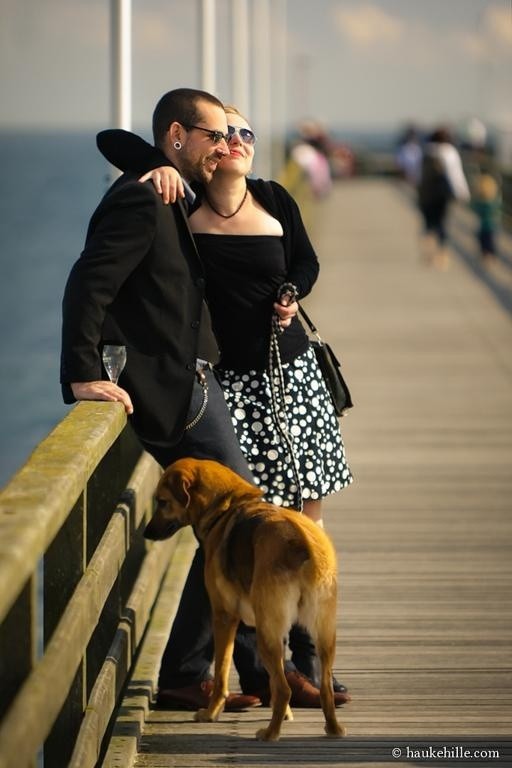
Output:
[202,182,248,220]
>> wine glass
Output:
[100,344,129,385]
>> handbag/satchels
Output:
[296,297,355,418]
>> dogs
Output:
[143,459,345,741]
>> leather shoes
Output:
[241,651,353,709]
[154,671,264,710]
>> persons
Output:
[92,106,350,709]
[58,86,350,713]
[282,115,470,274]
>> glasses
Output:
[180,123,233,145]
[227,124,257,144]
